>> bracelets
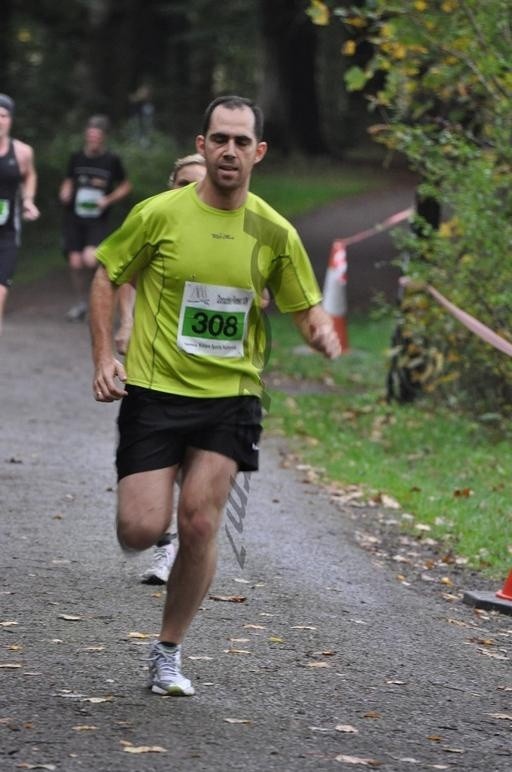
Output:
[19,192,35,200]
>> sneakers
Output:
[139,544,174,584]
[141,640,195,696]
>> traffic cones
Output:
[294,240,367,357]
[463,570,511,614]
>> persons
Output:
[0,92,44,322]
[84,91,347,699]
[107,149,273,586]
[58,113,131,326]
